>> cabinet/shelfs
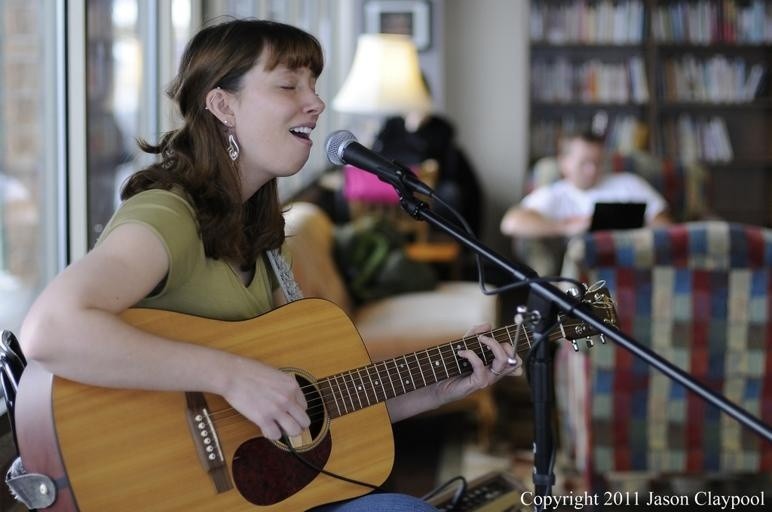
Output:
[527,0,771,224]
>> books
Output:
[529,0,772,171]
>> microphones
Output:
[324,130,435,201]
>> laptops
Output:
[589,202,647,229]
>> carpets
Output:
[434,405,543,511]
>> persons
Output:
[19,20,524,512]
[499,130,672,265]
[369,72,484,242]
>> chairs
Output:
[512,151,771,512]
[262,200,503,454]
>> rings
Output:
[489,367,502,377]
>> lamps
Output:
[329,31,433,144]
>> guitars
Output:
[5,279,623,512]
